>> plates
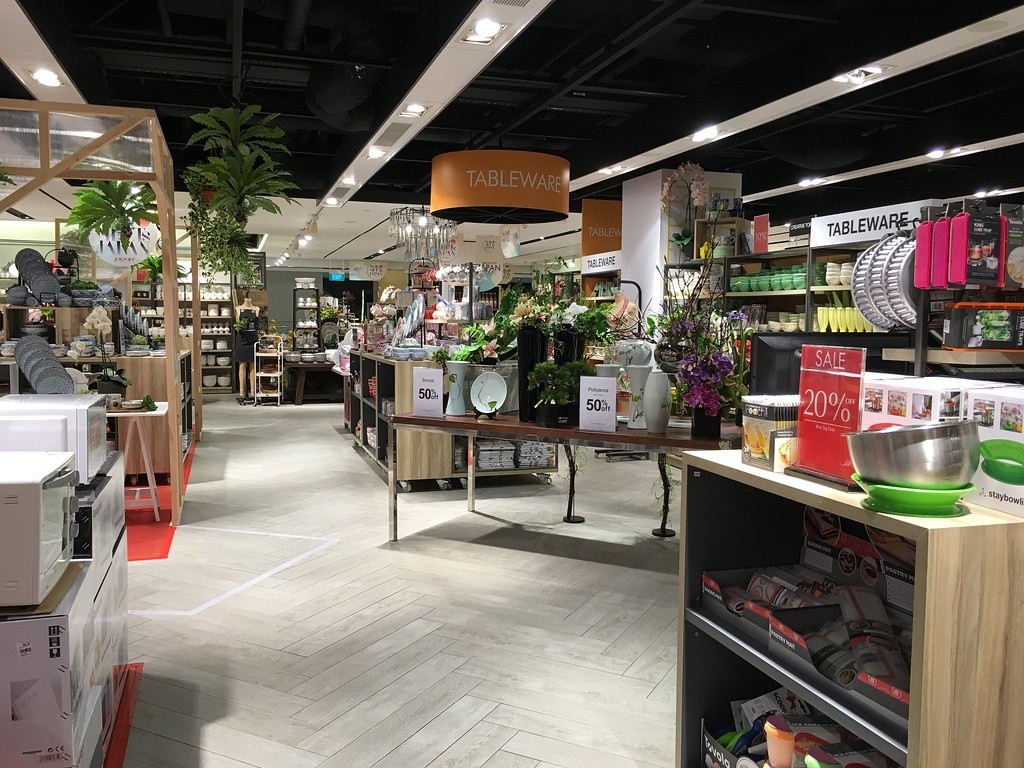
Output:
[392,294,425,346]
[14,335,88,394]
[125,349,166,357]
[470,372,508,414]
[15,248,61,302]
[121,300,148,344]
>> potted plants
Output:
[527,360,597,428]
[309,295,343,324]
[180,160,216,202]
[129,335,149,350]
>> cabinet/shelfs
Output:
[254,335,284,407]
[349,262,558,492]
[662,239,878,336]
[292,288,324,352]
[675,449,1024,768]
[0,306,195,485]
[131,281,236,394]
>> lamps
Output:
[275,214,319,267]
[389,205,458,262]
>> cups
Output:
[134,291,141,298]
[141,285,233,337]
[106,393,121,410]
[295,282,338,327]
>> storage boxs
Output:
[0,451,130,768]
[702,537,915,739]
[861,371,1024,517]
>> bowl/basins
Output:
[842,420,980,517]
[666,260,856,332]
[259,348,326,393]
[0,323,96,357]
[295,278,316,282]
[200,339,231,387]
[7,285,116,307]
[129,345,150,351]
[393,347,426,361]
[107,398,144,408]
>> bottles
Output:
[455,291,498,309]
[96,342,115,357]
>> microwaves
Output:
[0,393,107,606]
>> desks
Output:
[332,363,350,428]
[106,402,169,521]
[388,409,743,541]
[285,361,345,405]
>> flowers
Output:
[460,256,619,357]
[644,292,755,416]
[66,305,129,387]
[661,161,711,249]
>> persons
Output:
[234,297,260,398]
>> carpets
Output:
[124,425,196,561]
[104,663,144,768]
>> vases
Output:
[552,323,587,366]
[595,364,621,376]
[627,365,653,429]
[445,361,470,415]
[679,242,694,258]
[691,405,721,438]
[517,319,548,422]
[618,340,652,370]
[98,382,127,400]
